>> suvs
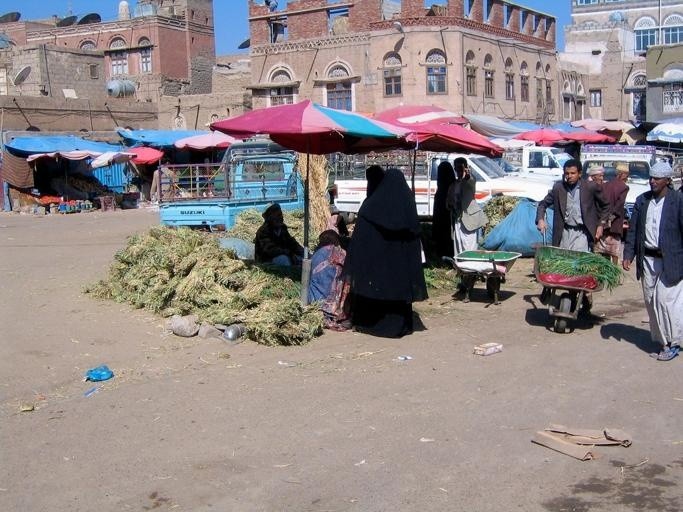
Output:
[216,142,336,202]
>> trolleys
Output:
[442,250,522,300]
[533,227,615,332]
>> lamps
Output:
[392,21,406,39]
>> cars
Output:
[579,152,656,230]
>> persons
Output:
[451,157,478,254]
[305,228,356,333]
[432,159,455,267]
[345,165,431,341]
[534,158,610,311]
[620,161,682,362]
[255,202,305,268]
[591,161,631,267]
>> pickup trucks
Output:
[332,152,554,223]
[493,158,561,185]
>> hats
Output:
[616,162,630,172]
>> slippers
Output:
[658,346,679,360]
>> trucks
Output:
[508,146,575,183]
[160,154,309,233]
